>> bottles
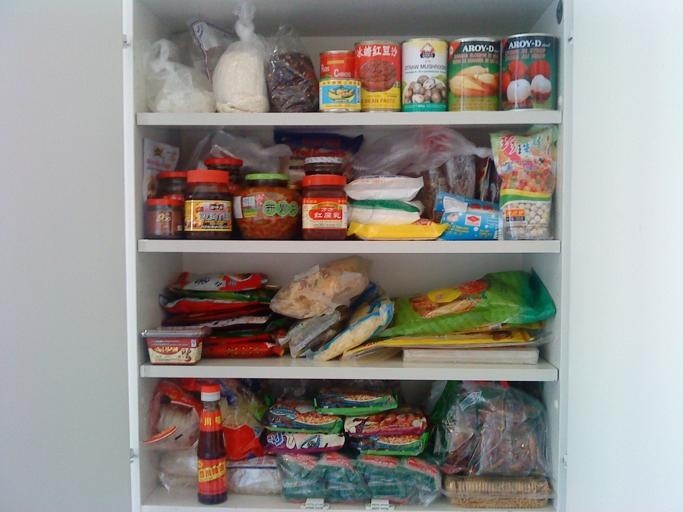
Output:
[196,380,229,507]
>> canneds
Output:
[145,156,348,240]
[319,32,558,112]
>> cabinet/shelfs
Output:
[119,1,574,510]
